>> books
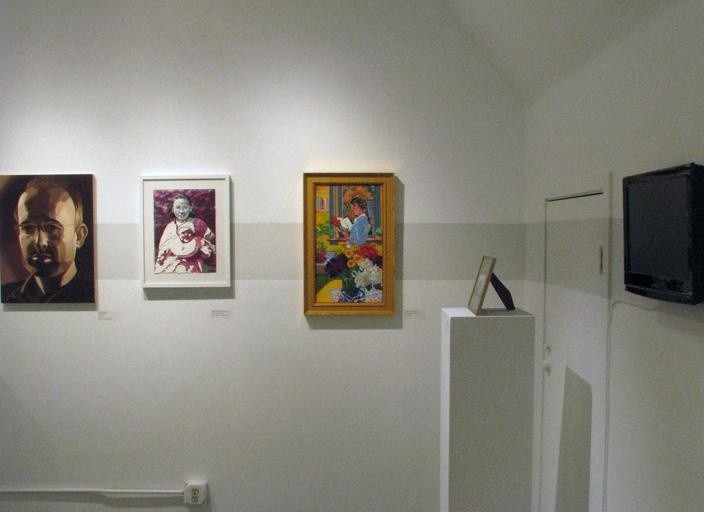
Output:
[337,215,353,233]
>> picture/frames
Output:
[304,171,397,315]
[467,255,515,315]
[140,175,231,289]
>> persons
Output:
[158,222,211,274]
[1,175,94,303]
[154,190,216,275]
[325,193,373,281]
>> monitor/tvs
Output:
[622,163,704,305]
